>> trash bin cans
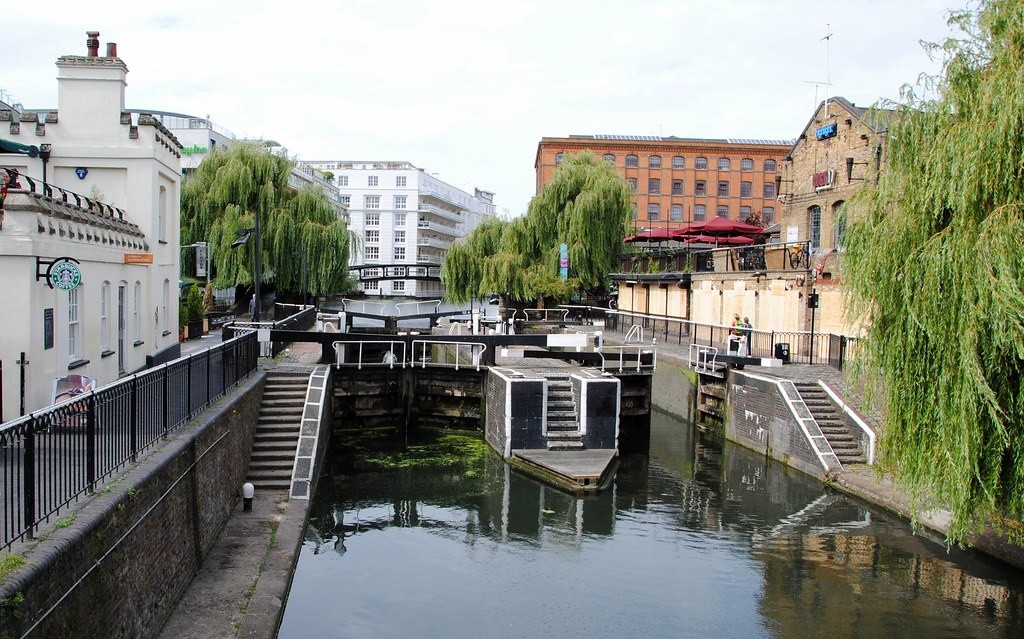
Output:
[576,311,583,321]
[775,343,790,364]
[188,318,203,340]
[607,313,617,329]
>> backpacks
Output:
[735,320,743,334]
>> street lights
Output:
[230,226,260,329]
[190,245,211,283]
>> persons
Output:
[201,291,206,299]
[731,313,744,336]
[249,294,261,327]
[743,317,753,357]
[1,169,21,196]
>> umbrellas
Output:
[623,217,761,249]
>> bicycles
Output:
[790,244,811,269]
